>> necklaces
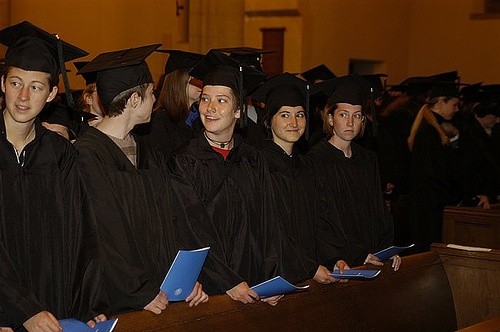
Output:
[204,131,233,148]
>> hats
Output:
[212,47,274,64]
[188,50,267,129]
[321,75,380,137]
[392,71,500,114]
[73,61,97,86]
[38,100,98,141]
[76,44,163,112]
[251,72,322,141]
[301,65,337,82]
[368,74,389,99]
[154,50,205,75]
[0,21,90,108]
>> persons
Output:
[0,22,500,332]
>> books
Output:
[58,318,95,332]
[330,269,381,278]
[250,276,310,299]
[160,247,211,301]
[92,318,118,332]
[373,244,415,261]
